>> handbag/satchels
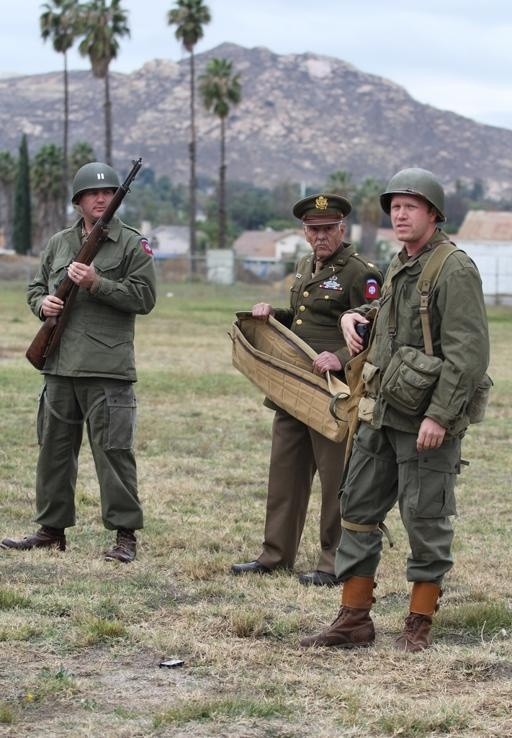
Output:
[469,372,492,423]
[381,345,441,417]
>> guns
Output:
[26,158,143,370]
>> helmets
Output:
[380,167,446,221]
[71,163,121,202]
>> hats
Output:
[293,193,351,226]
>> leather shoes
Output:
[299,569,340,587]
[0,527,67,552]
[229,562,267,574]
[106,535,138,564]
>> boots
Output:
[395,613,432,654]
[299,607,375,646]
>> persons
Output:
[0,162,156,563]
[231,195,385,588]
[299,167,489,654]
